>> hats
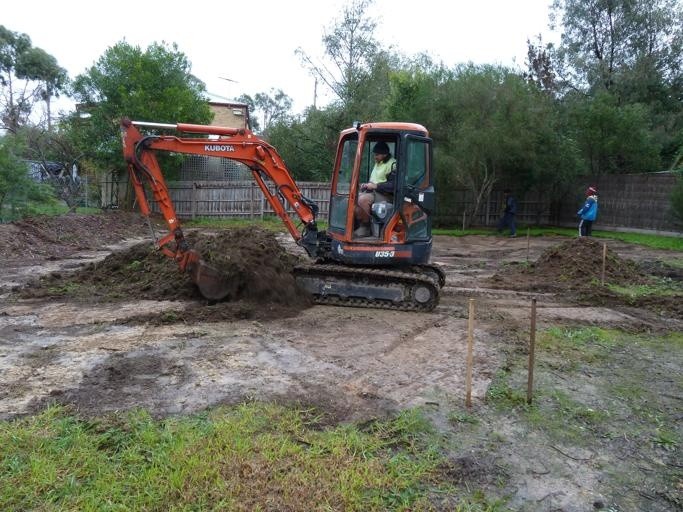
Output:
[372,141,389,154]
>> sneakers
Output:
[353,225,371,237]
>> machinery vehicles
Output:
[111,112,448,312]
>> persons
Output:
[496,188,518,238]
[353,140,397,238]
[575,182,599,237]
